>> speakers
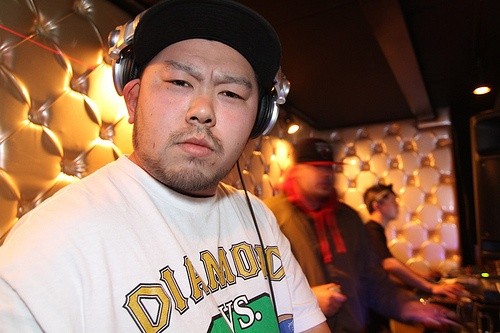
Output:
[469,105,499,280]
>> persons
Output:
[0,0,333,332]
[361,184,464,332]
[261,137,461,333]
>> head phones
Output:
[106,6,291,139]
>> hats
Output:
[131,0,282,102]
[294,137,347,166]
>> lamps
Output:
[284,113,300,136]
